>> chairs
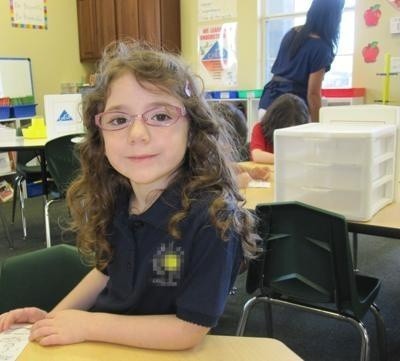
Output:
[236,201,387,361]
[0,134,93,313]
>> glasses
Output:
[90,105,189,130]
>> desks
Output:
[236,160,400,272]
[14,335,305,361]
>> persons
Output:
[210,100,273,188]
[258,0,344,124]
[0,35,264,347]
[249,94,311,166]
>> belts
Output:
[270,74,295,85]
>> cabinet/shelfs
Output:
[76,0,181,64]
[272,121,397,222]
